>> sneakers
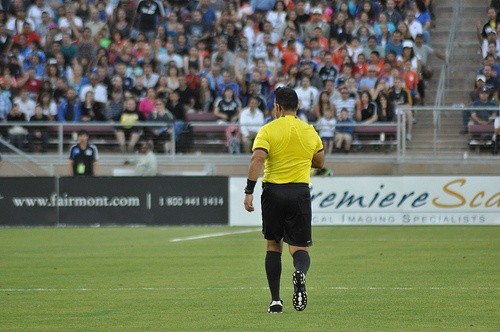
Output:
[292,270,308,311]
[267,300,283,313]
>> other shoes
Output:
[406,133,412,140]
[413,118,416,123]
[335,148,341,152]
[344,150,349,153]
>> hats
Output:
[417,34,422,37]
[479,87,489,92]
[476,74,486,82]
[403,39,414,48]
[485,27,496,34]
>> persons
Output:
[0,0,447,177]
[461,0,500,155]
[243,87,325,313]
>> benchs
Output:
[30,108,398,157]
[467,123,500,155]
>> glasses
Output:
[273,87,281,104]
[407,15,414,17]
